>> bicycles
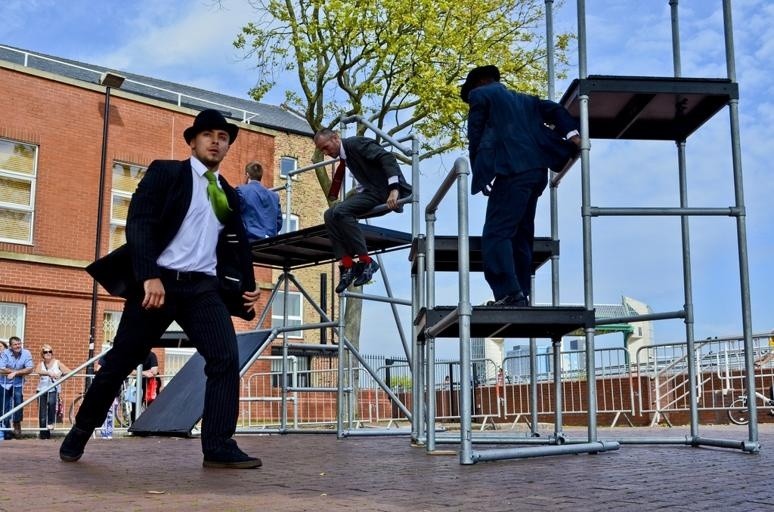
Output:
[69,394,128,428]
[727,362,774,425]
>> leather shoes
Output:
[488,293,528,307]
[202,438,262,468]
[60,426,94,462]
[354,258,379,286]
[336,261,355,292]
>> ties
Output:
[328,158,346,202]
[205,172,233,222]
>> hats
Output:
[461,65,500,103]
[184,109,238,144]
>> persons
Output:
[0,336,73,440]
[94,341,159,427]
[314,126,413,292]
[233,160,284,246]
[460,64,592,308]
[443,376,450,391]
[58,110,262,467]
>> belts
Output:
[162,268,207,281]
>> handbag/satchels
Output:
[57,397,64,417]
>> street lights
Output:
[81,72,126,394]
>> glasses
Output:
[43,350,52,354]
